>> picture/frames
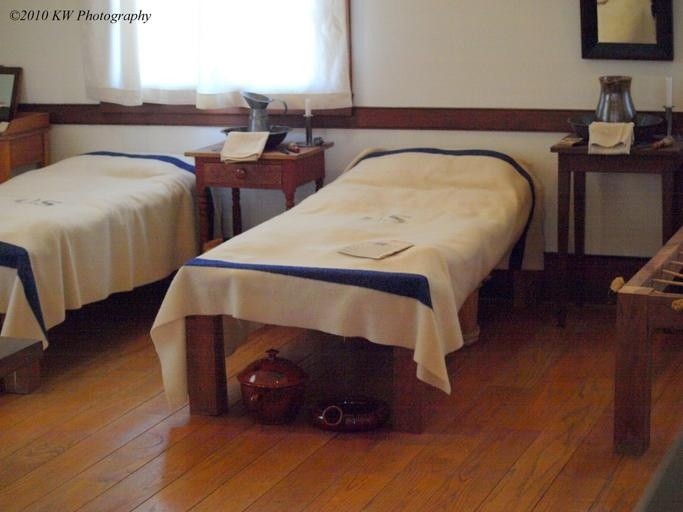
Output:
[581,1,673,61]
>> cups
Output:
[592,76,637,123]
[240,90,288,132]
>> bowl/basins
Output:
[567,113,664,146]
[221,126,291,151]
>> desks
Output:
[183,136,330,253]
[0,111,52,183]
[550,130,682,330]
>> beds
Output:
[148,146,546,436]
[0,150,226,399]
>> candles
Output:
[663,76,674,106]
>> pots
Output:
[235,348,309,425]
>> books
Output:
[338,239,413,259]
